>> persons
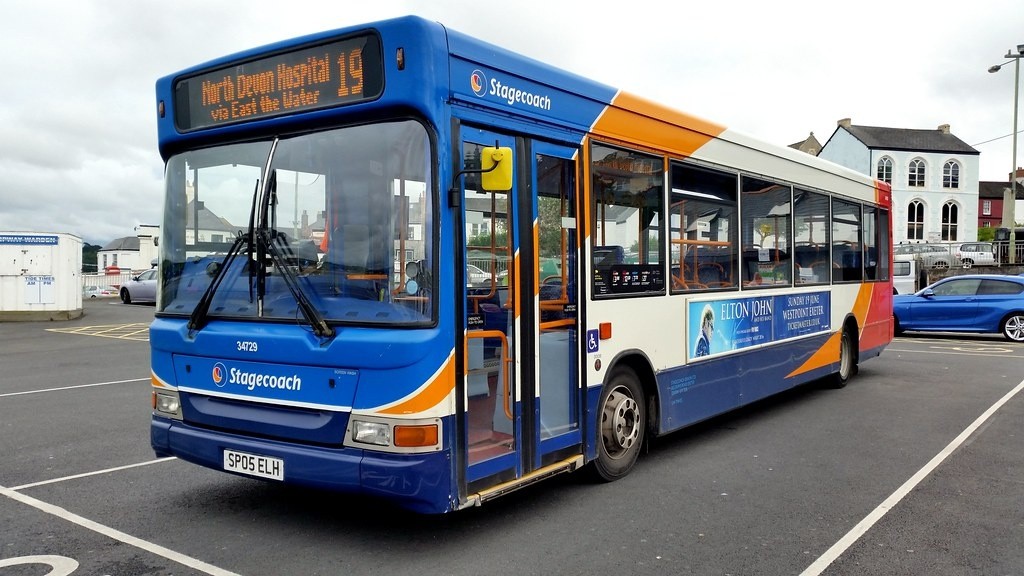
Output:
[694,305,714,358]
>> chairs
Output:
[663,240,879,290]
[538,245,625,329]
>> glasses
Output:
[708,320,714,331]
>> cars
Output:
[119,269,158,304]
[947,242,1000,269]
[892,274,1024,342]
[466,264,498,287]
[82,285,120,299]
[892,245,952,273]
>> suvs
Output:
[991,227,1024,265]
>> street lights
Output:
[988,44,1024,263]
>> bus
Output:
[149,15,894,514]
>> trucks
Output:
[892,260,973,295]
